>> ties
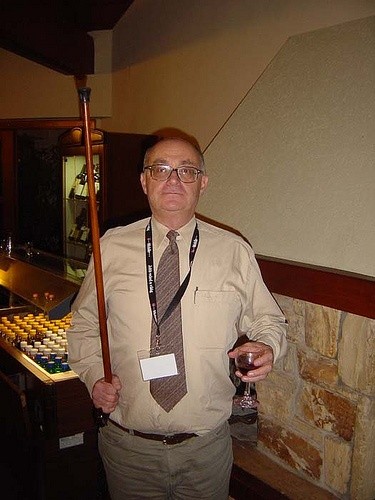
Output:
[150,229,188,414]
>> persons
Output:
[66,133,288,500]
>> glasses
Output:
[144,163,203,184]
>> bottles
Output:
[0,313,72,373]
[69,207,90,243]
[68,164,100,200]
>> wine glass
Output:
[234,346,265,408]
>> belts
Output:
[108,418,198,444]
